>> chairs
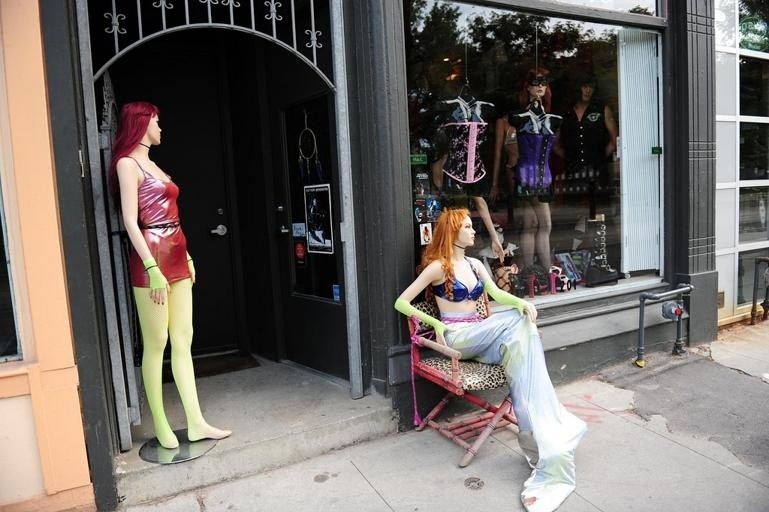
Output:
[408,302,526,468]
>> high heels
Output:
[528,264,566,299]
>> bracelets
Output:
[187,258,193,261]
[144,265,160,270]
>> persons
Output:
[395,207,586,512]
[427,65,619,293]
[107,101,232,449]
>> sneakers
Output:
[584,266,625,287]
